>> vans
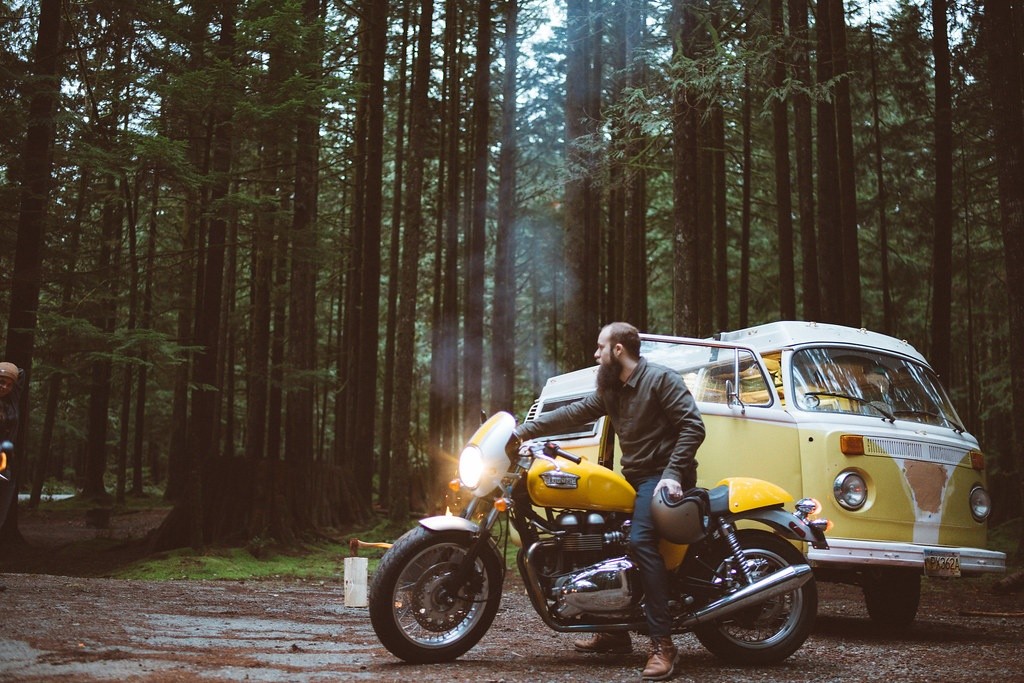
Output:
[509,321,1006,627]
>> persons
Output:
[0,362,26,591]
[512,322,706,679]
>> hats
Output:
[0,361,19,383]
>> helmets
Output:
[651,486,713,545]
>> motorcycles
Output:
[366,411,834,665]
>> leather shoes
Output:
[642,634,680,681]
[573,628,635,654]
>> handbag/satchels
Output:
[341,554,370,608]
